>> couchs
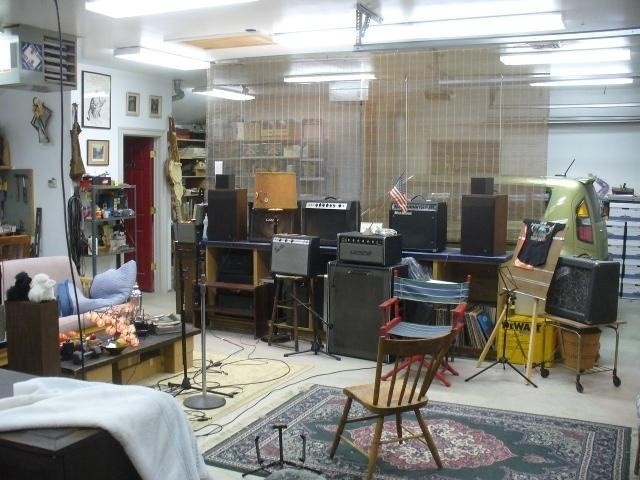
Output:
[2,258,142,348]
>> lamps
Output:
[252,169,299,245]
[82,6,635,105]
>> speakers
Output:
[301,198,360,245]
[390,201,448,253]
[271,233,320,276]
[544,257,620,325]
[215,174,236,189]
[249,207,307,248]
[460,196,509,257]
[207,188,247,242]
[327,260,409,371]
[337,231,403,267]
[470,175,494,195]
[5,299,59,381]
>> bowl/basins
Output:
[102,341,129,354]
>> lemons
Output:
[115,338,125,348]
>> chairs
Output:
[329,329,459,479]
[1,235,31,261]
[378,273,473,386]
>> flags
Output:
[388,177,407,214]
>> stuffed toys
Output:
[27,272,57,302]
[6,271,32,302]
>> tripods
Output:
[283,284,341,365]
[464,300,538,387]
[163,255,234,401]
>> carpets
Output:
[199,385,640,480]
[135,348,315,433]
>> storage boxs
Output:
[603,203,640,301]
[230,119,294,141]
[496,313,556,368]
[173,223,204,244]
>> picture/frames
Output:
[147,94,163,119]
[80,70,113,129]
[125,89,141,118]
[86,139,110,167]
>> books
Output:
[149,312,182,334]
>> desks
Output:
[0,366,134,478]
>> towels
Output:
[1,378,210,480]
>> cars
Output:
[385,157,610,261]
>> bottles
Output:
[85,206,134,256]
[130,281,142,317]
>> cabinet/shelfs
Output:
[67,174,140,289]
[172,240,205,324]
[199,239,513,363]
[175,133,325,200]
